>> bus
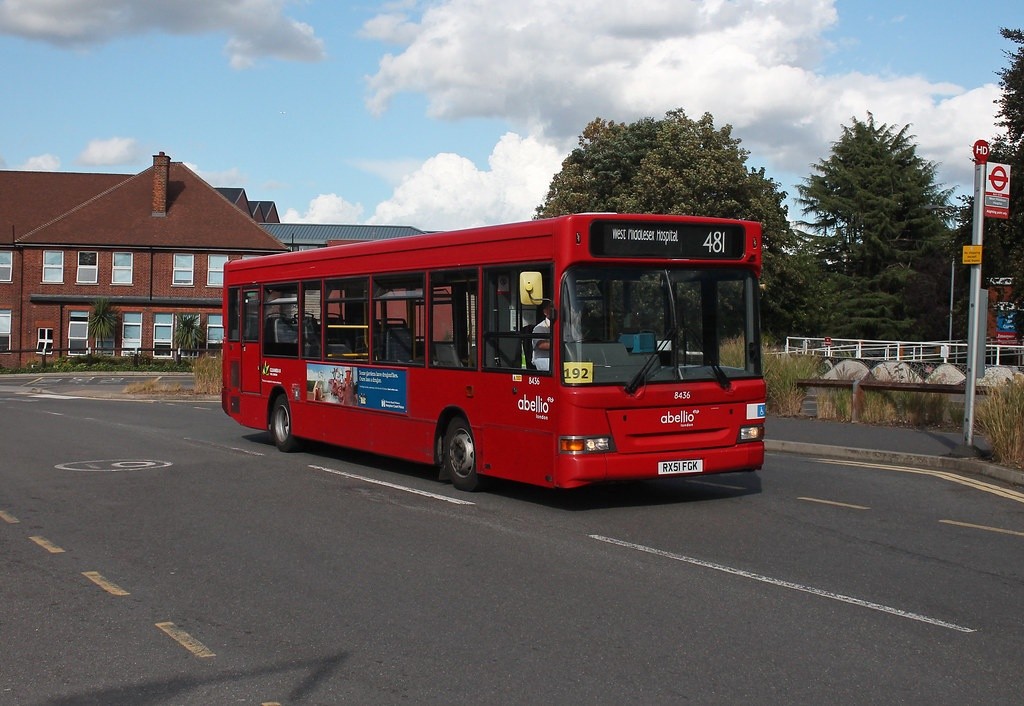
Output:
[222,212,769,491]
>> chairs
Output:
[432,341,456,367]
[264,312,424,363]
[519,325,536,370]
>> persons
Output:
[531,303,581,372]
